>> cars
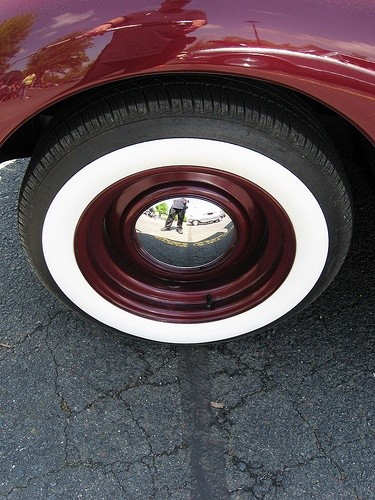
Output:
[188,207,225,226]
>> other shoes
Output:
[162,227,170,231]
[177,228,183,234]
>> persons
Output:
[159,197,189,235]
